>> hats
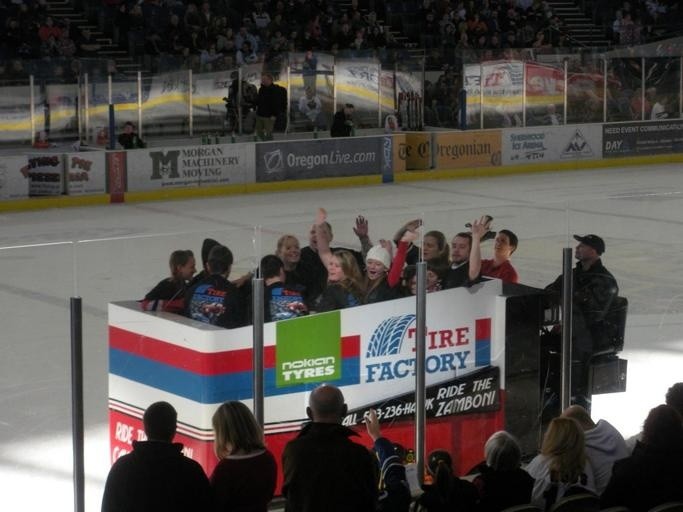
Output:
[367,245,391,272]
[572,234,604,255]
[202,239,220,258]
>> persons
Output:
[208,401,277,512]
[281,385,379,512]
[667,383,683,417]
[409,449,481,512]
[597,404,683,512]
[101,402,209,512]
[561,404,631,497]
[541,234,619,413]
[524,416,597,511]
[1,0,682,147]
[364,408,411,512]
[471,430,534,512]
[142,207,518,327]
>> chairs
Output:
[583,295,628,366]
[503,504,545,512]
[550,494,602,512]
[647,502,683,512]
[599,506,630,512]
